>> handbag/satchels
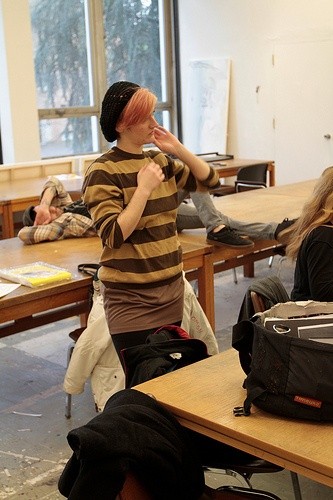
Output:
[77,262,102,310]
[232,315,333,425]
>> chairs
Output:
[234,274,289,325]
[122,341,302,500]
[58,388,281,500]
[209,162,274,285]
[63,284,220,421]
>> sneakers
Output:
[274,216,303,244]
[206,225,255,248]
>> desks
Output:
[0,153,333,500]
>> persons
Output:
[17,178,299,247]
[286,166,333,302]
[81,82,221,373]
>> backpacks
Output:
[121,328,254,481]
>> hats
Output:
[23,205,35,227]
[100,81,141,142]
